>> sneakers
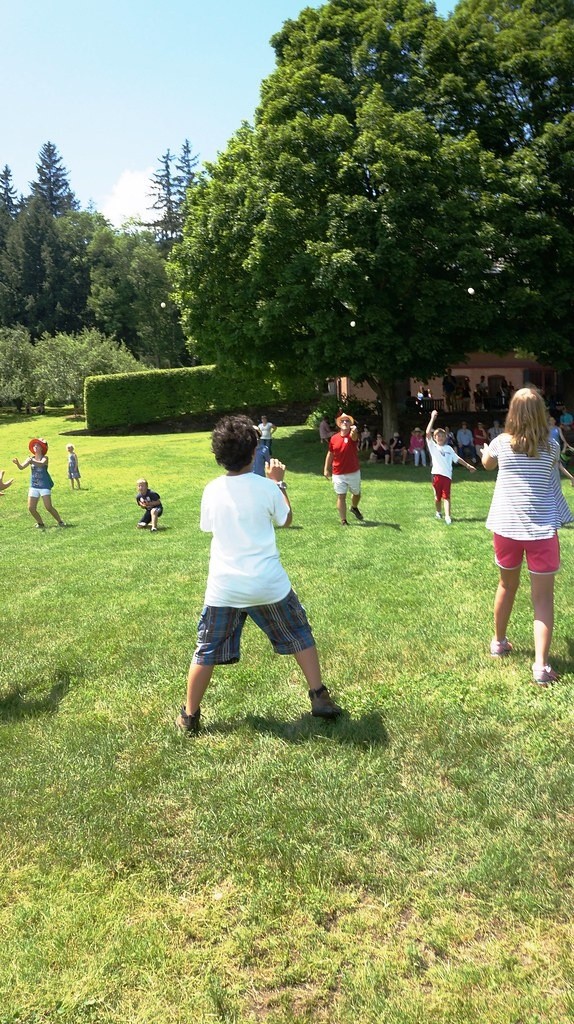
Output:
[176,706,202,730]
[308,684,343,716]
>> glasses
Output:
[340,420,350,423]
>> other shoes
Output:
[436,510,443,520]
[341,519,349,526]
[445,515,452,525]
[349,506,363,520]
[490,640,512,655]
[533,666,559,684]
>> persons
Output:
[173,415,342,734]
[0,471,14,497]
[13,438,65,529]
[135,479,163,532]
[318,366,574,524]
[479,386,574,684]
[67,443,81,491]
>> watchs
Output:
[276,480,287,490]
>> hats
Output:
[377,434,383,438]
[394,432,400,437]
[28,438,49,455]
[411,427,425,435]
[336,413,354,429]
